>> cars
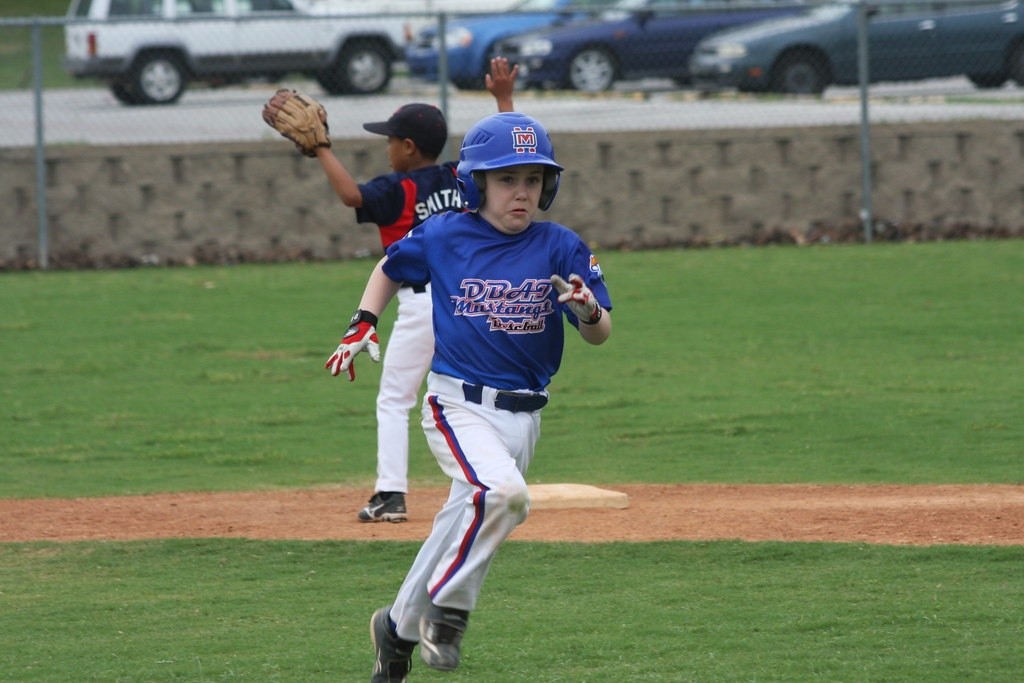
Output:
[405,0,618,90]
[489,0,818,94]
[688,0,1024,98]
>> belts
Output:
[413,282,426,294]
[462,383,549,413]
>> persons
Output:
[326,112,616,682]
[263,55,517,522]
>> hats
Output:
[363,103,447,154]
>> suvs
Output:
[62,0,413,106]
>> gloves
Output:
[324,309,381,381]
[550,272,603,325]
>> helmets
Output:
[454,111,565,213]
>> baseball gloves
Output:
[261,87,332,158]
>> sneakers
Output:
[369,606,420,683]
[418,602,469,671]
[357,491,407,523]
